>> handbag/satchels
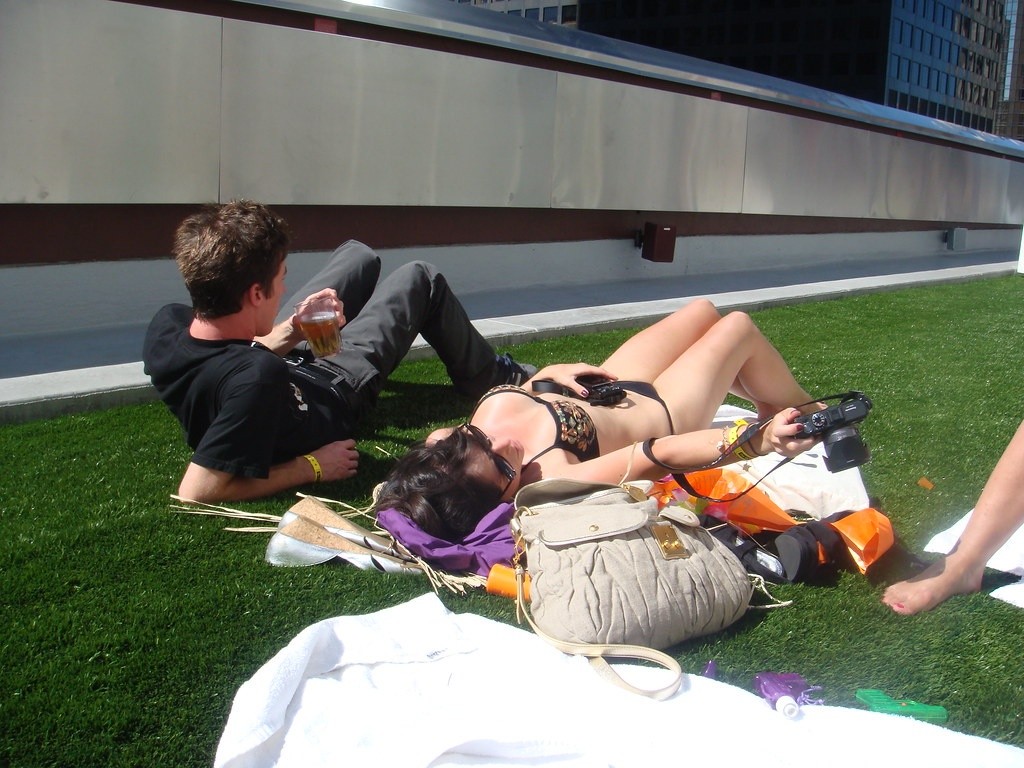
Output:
[514,476,751,648]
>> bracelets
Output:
[717,419,768,463]
[303,454,323,483]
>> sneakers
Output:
[461,353,536,405]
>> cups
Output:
[292,295,342,360]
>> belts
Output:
[282,356,364,419]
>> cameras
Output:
[793,395,871,474]
[576,374,628,406]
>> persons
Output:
[374,298,829,542]
[880,418,1024,617]
[141,200,538,504]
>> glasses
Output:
[446,423,517,498]
